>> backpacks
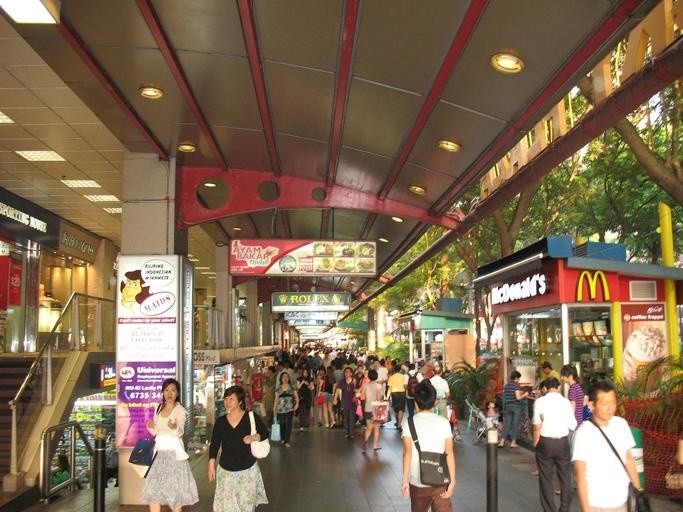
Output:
[406,372,421,398]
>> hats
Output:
[540,361,553,367]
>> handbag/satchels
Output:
[127,431,160,480]
[248,410,271,459]
[355,399,363,417]
[626,482,653,512]
[316,394,326,405]
[371,398,390,424]
[418,451,452,486]
[270,414,281,442]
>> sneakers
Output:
[298,421,355,439]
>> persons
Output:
[268,341,449,453]
[571,375,641,511]
[555,365,584,493]
[105,435,118,488]
[400,381,457,512]
[139,378,200,512]
[206,385,269,512]
[533,377,578,512]
[487,362,559,448]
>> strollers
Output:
[463,398,503,446]
